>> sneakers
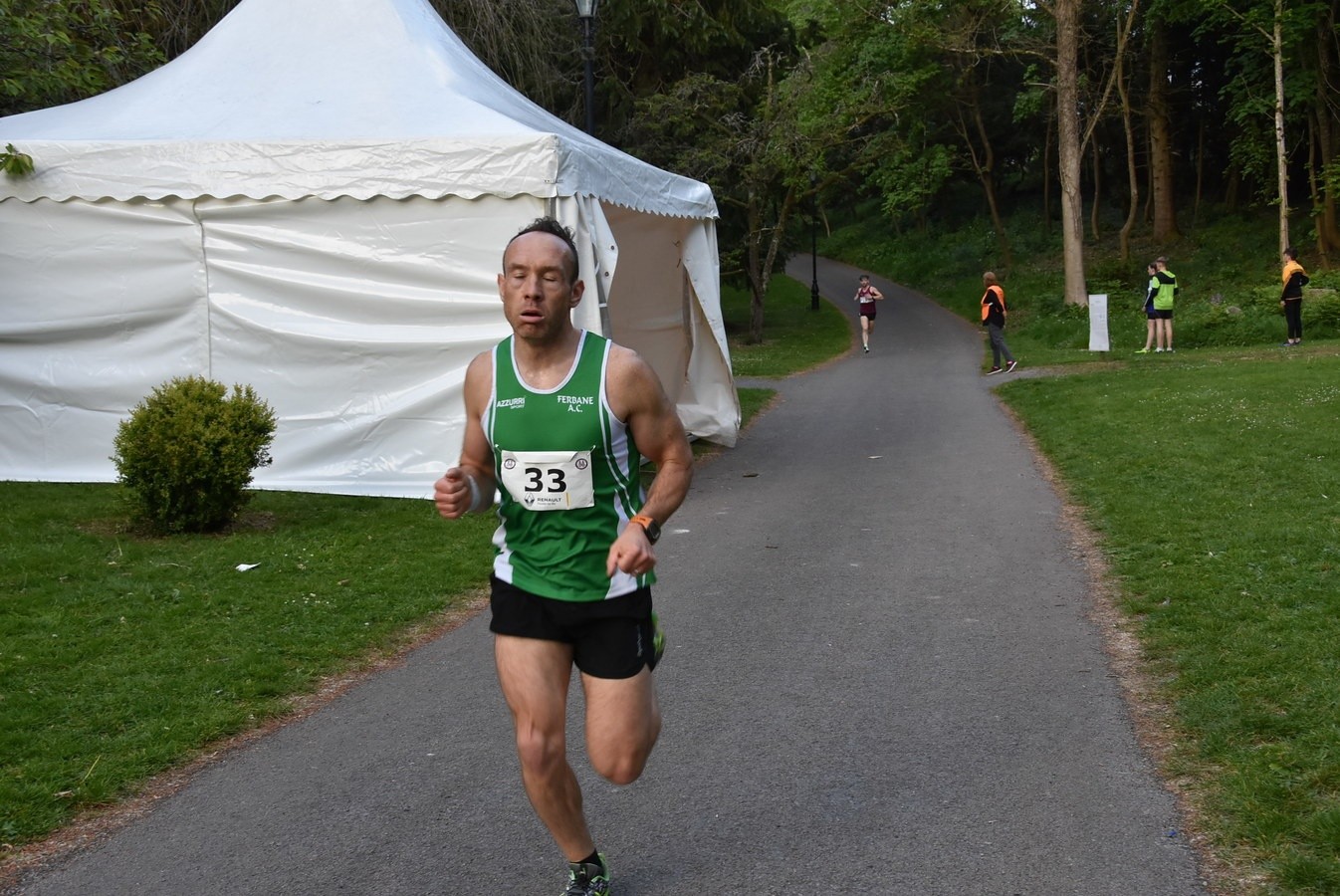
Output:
[987,367,1002,375]
[652,611,665,662]
[559,853,611,896]
[1005,361,1017,374]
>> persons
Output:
[1135,256,1179,353]
[980,272,1017,376]
[1278,246,1310,348]
[435,217,694,896]
[853,275,885,354]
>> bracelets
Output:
[467,475,481,513]
[872,295,874,300]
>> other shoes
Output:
[1134,349,1150,353]
[1282,341,1296,346]
[864,346,869,352]
[1153,349,1163,354]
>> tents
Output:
[1,0,742,501]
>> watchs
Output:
[628,513,663,548]
